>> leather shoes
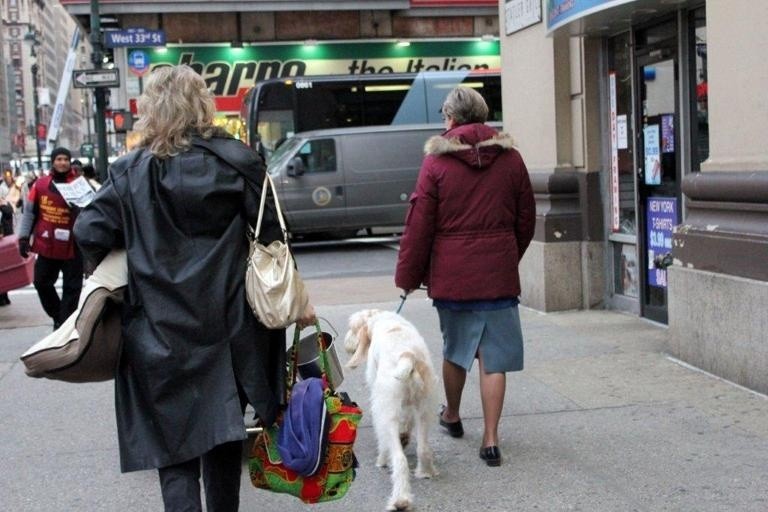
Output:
[479,446,500,466]
[438,403,465,437]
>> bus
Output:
[241,68,501,239]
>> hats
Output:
[51,148,72,166]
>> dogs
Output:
[344,308,440,512]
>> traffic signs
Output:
[72,69,120,88]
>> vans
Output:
[21,156,51,178]
[265,120,503,234]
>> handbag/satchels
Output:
[248,316,364,504]
[244,172,310,331]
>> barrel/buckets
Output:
[284,317,344,391]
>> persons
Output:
[0,147,103,331]
[70,64,317,510]
[393,85,537,467]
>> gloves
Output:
[19,236,32,259]
